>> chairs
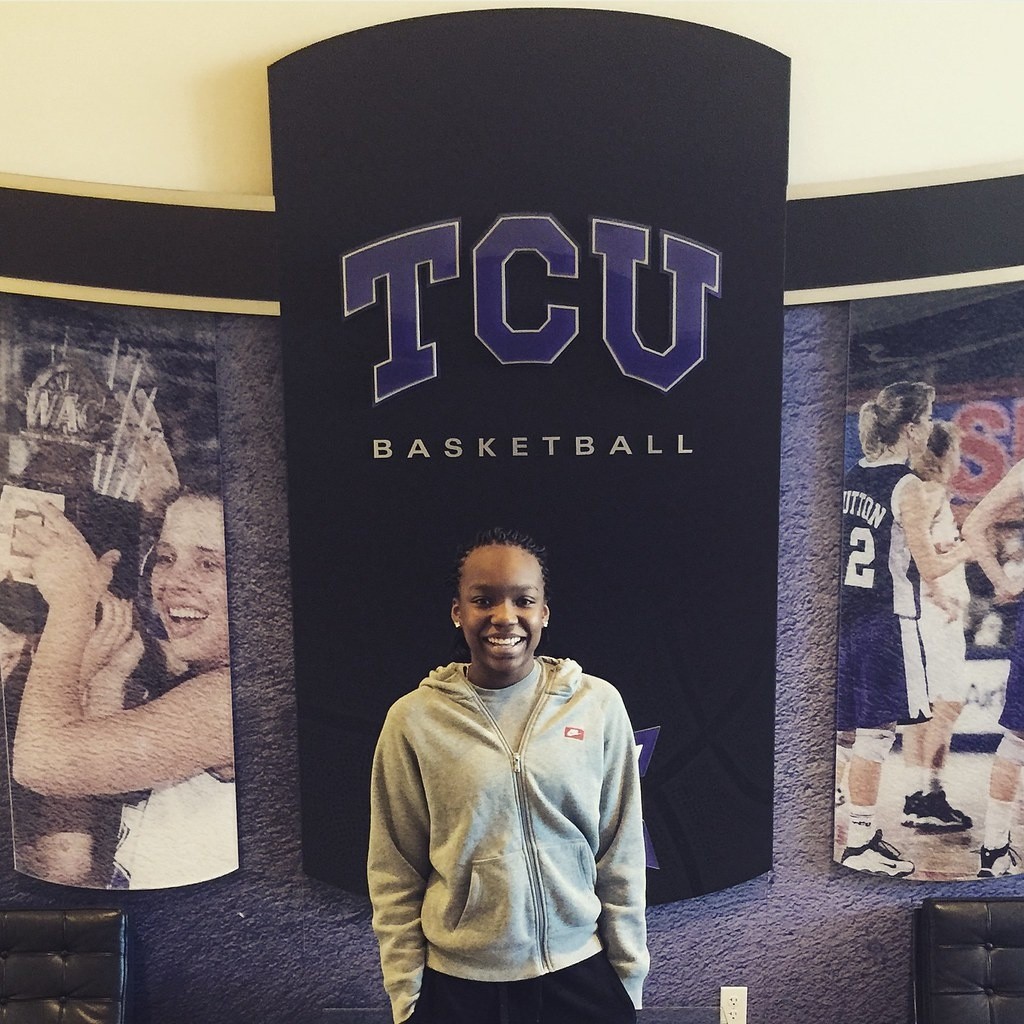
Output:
[0,906,134,1024]
[909,898,1024,1024]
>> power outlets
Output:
[720,986,748,1024]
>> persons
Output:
[0,477,238,891]
[835,382,1024,878]
[367,527,650,1024]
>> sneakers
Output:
[900,790,972,835]
[839,829,915,877]
[978,832,1023,880]
